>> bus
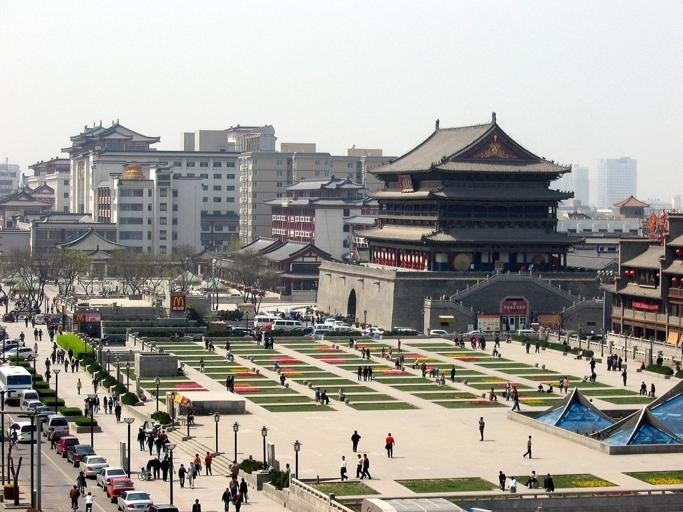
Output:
[0,365,32,402]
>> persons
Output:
[49,428,57,450]
[321,390,331,405]
[226,375,235,392]
[20,311,80,382]
[498,470,555,493]
[51,297,60,314]
[339,430,372,481]
[77,377,121,421]
[170,331,183,341]
[338,387,344,401]
[478,416,485,442]
[279,372,286,385]
[315,387,321,400]
[273,363,279,372]
[254,327,274,349]
[137,420,250,512]
[482,383,521,412]
[200,358,205,371]
[280,305,356,326]
[413,357,456,386]
[522,435,532,459]
[285,463,290,475]
[205,339,234,362]
[69,471,94,511]
[382,339,405,372]
[385,433,395,458]
[358,365,372,382]
[351,338,371,359]
[521,320,663,398]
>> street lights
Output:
[290,440,302,479]
[245,311,249,332]
[170,391,175,428]
[212,413,219,453]
[86,393,96,449]
[122,417,134,478]
[153,376,161,410]
[558,320,654,366]
[184,311,189,335]
[363,310,366,331]
[231,422,239,463]
[1,325,60,415]
[162,443,176,504]
[186,399,191,436]
[82,335,130,393]
[258,425,268,469]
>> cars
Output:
[20,389,178,512]
[100,327,256,345]
[0,339,37,361]
[314,319,603,340]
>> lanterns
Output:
[671,276,677,283]
[676,249,683,256]
[629,269,635,275]
[624,268,629,275]
[680,277,683,283]
[656,272,660,278]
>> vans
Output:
[6,422,37,442]
[270,320,304,332]
[254,315,284,328]
[35,314,45,325]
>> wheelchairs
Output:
[137,468,152,481]
